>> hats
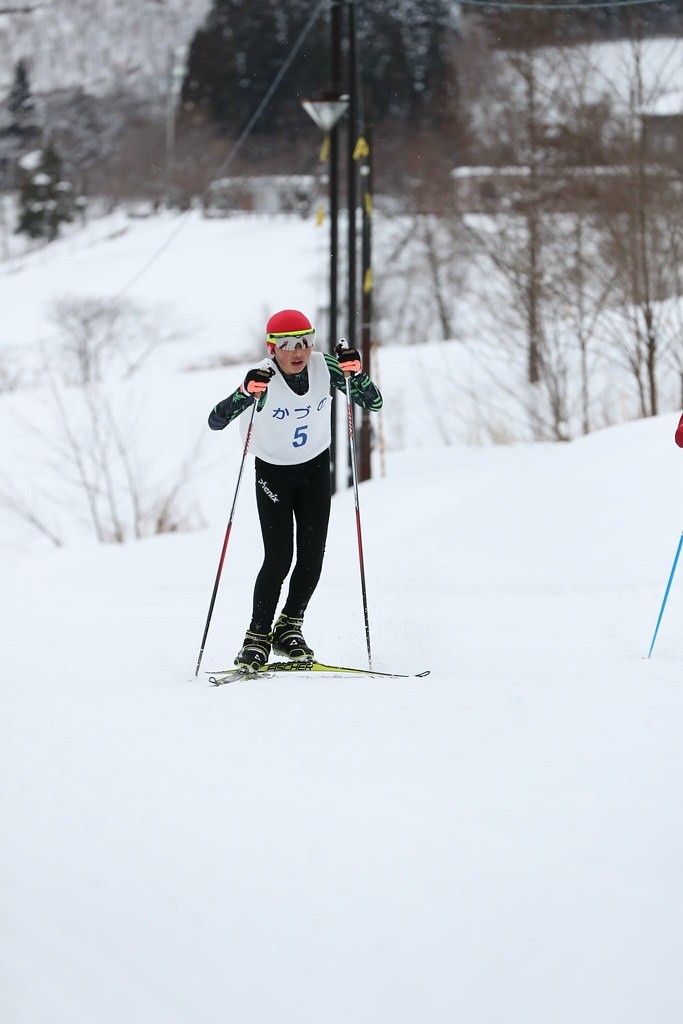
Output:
[266,310,312,355]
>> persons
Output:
[675,411,683,449]
[208,310,383,671]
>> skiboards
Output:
[202,658,433,686]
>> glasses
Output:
[276,334,315,351]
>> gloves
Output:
[334,347,362,377]
[241,367,276,396]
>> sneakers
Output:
[234,630,274,673]
[273,613,314,660]
